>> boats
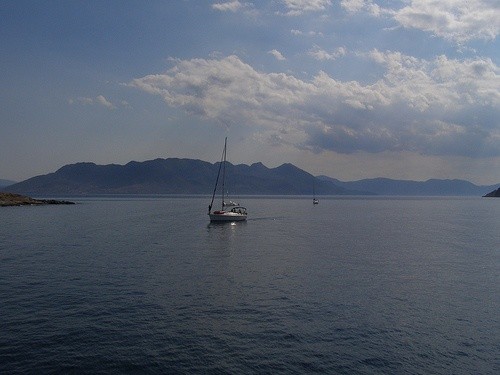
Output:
[207,137,248,222]
[313,184,319,204]
